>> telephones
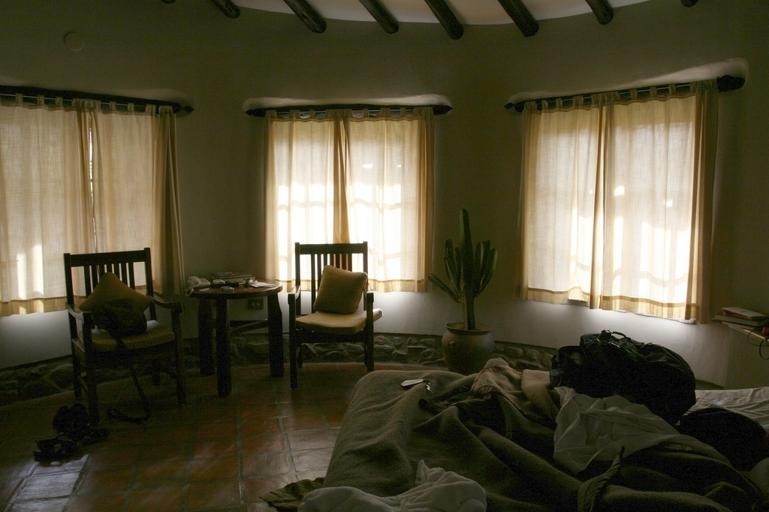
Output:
[98,299,146,337]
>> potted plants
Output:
[423,204,498,373]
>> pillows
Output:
[96,301,147,334]
[310,264,368,312]
[77,271,154,314]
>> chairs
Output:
[63,246,188,424]
[288,244,384,392]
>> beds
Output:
[263,358,768,512]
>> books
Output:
[711,303,765,333]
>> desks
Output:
[188,277,286,399]
